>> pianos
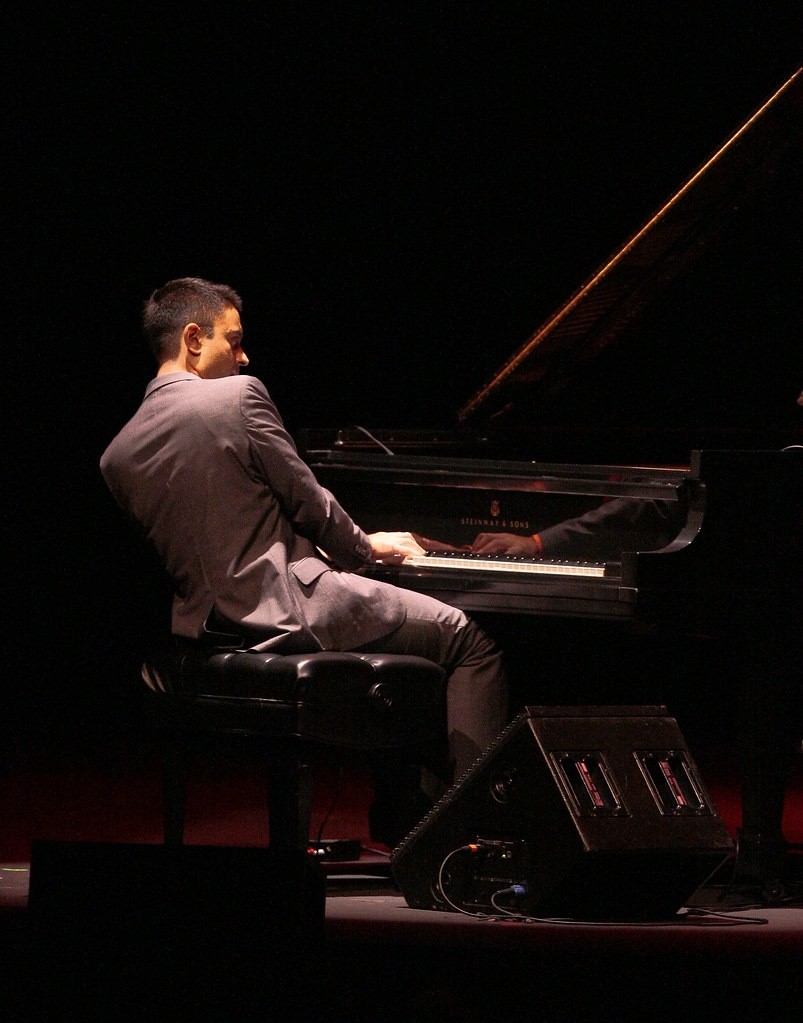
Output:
[301,445,712,620]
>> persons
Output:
[100,275,513,876]
[466,496,678,561]
